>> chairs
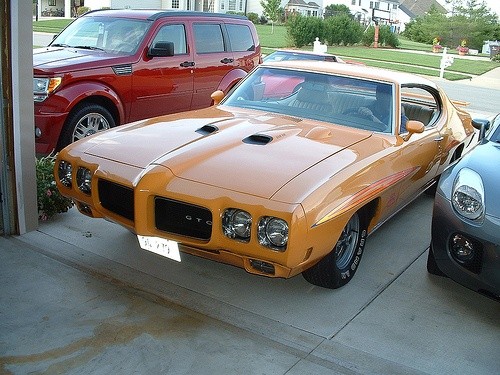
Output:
[289,89,332,113]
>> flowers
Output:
[432,37,442,49]
[455,39,469,52]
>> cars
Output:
[260,50,366,101]
[427,111,500,306]
[52,59,477,290]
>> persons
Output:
[356,84,409,133]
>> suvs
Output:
[32,7,262,160]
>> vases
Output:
[458,51,467,56]
[432,47,439,52]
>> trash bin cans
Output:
[489,44,499,59]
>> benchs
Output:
[328,92,436,124]
